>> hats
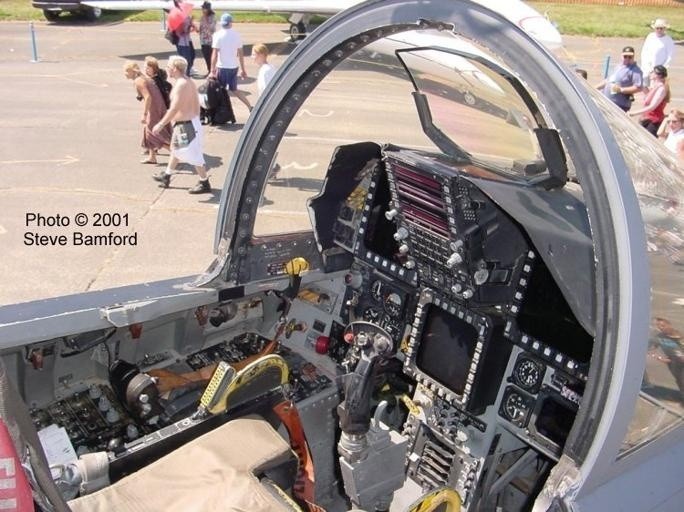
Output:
[651,19,670,29]
[220,14,232,25]
[202,2,211,8]
[622,47,634,56]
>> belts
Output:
[173,121,192,128]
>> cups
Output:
[610,80,618,95]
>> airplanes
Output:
[81,0,587,113]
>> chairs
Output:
[0,359,311,512]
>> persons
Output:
[626,64,670,139]
[593,45,642,112]
[123,0,281,195]
[655,109,683,160]
[638,18,673,103]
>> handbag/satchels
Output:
[165,29,178,45]
[197,78,236,125]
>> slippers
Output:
[140,159,156,164]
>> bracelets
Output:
[621,87,624,94]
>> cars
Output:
[32,1,103,21]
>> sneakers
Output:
[141,150,158,155]
[190,181,212,195]
[152,172,172,189]
